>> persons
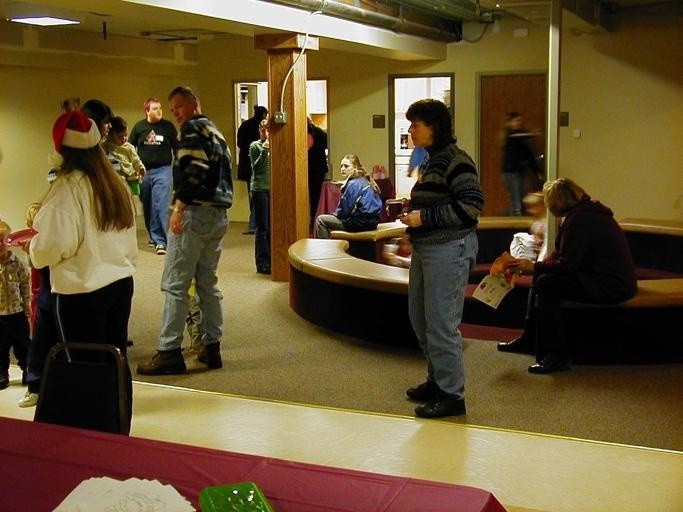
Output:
[1,86,233,406]
[521,191,547,257]
[313,152,384,238]
[494,110,546,218]
[238,105,270,275]
[496,177,638,375]
[384,98,484,420]
[405,145,428,178]
[306,116,329,230]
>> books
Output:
[471,251,523,311]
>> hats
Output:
[53,111,102,152]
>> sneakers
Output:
[135,341,222,376]
[1,368,39,408]
[148,239,166,254]
[406,380,466,418]
[242,230,255,234]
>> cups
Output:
[387,200,403,218]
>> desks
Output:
[311,179,396,240]
[0,416,509,512]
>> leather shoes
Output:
[498,334,544,355]
[528,352,564,373]
